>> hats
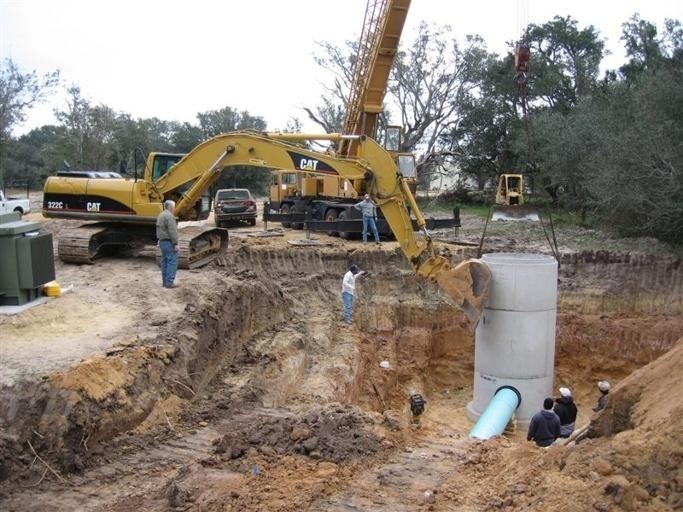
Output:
[597,381,611,391]
[559,386,572,396]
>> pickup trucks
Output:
[0,188,31,218]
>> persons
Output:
[592,381,611,412]
[354,194,383,246]
[553,387,577,439]
[341,264,365,324]
[527,398,560,447]
[156,200,182,288]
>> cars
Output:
[213,188,258,227]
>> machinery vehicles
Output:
[261,0,531,241]
[42,128,493,336]
